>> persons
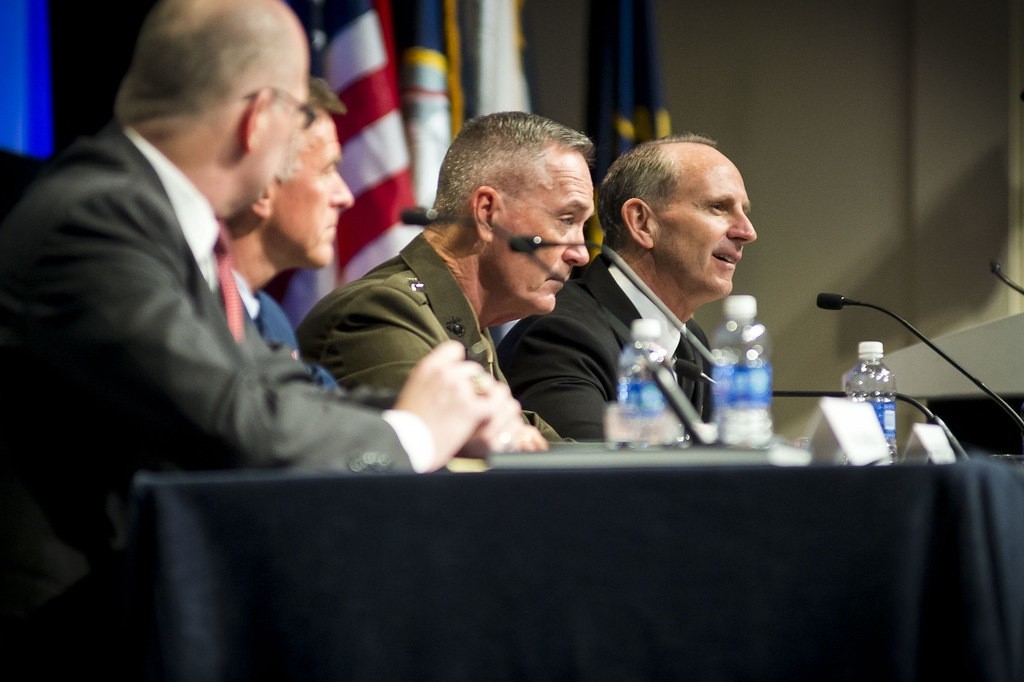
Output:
[295,111,595,456]
[218,76,547,453]
[497,135,757,444]
[0,0,522,474]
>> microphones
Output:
[399,206,1024,464]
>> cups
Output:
[600,401,643,453]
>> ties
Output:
[212,227,249,348]
[675,331,701,418]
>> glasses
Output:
[241,84,317,131]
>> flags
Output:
[583,0,672,263]
[283,0,542,294]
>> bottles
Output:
[616,318,680,446]
[710,295,775,444]
[842,342,900,466]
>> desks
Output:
[96,451,1024,681]
